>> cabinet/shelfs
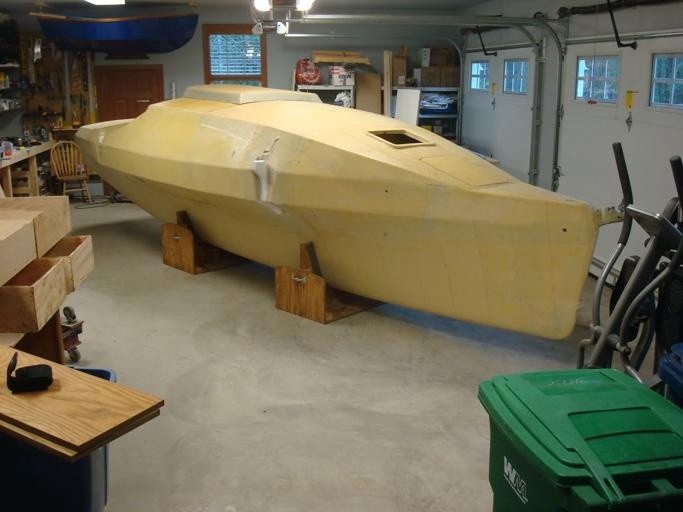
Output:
[0,195,97,365]
[291,69,462,145]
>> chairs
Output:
[49,139,93,202]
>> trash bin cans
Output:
[478,369,683,511]
